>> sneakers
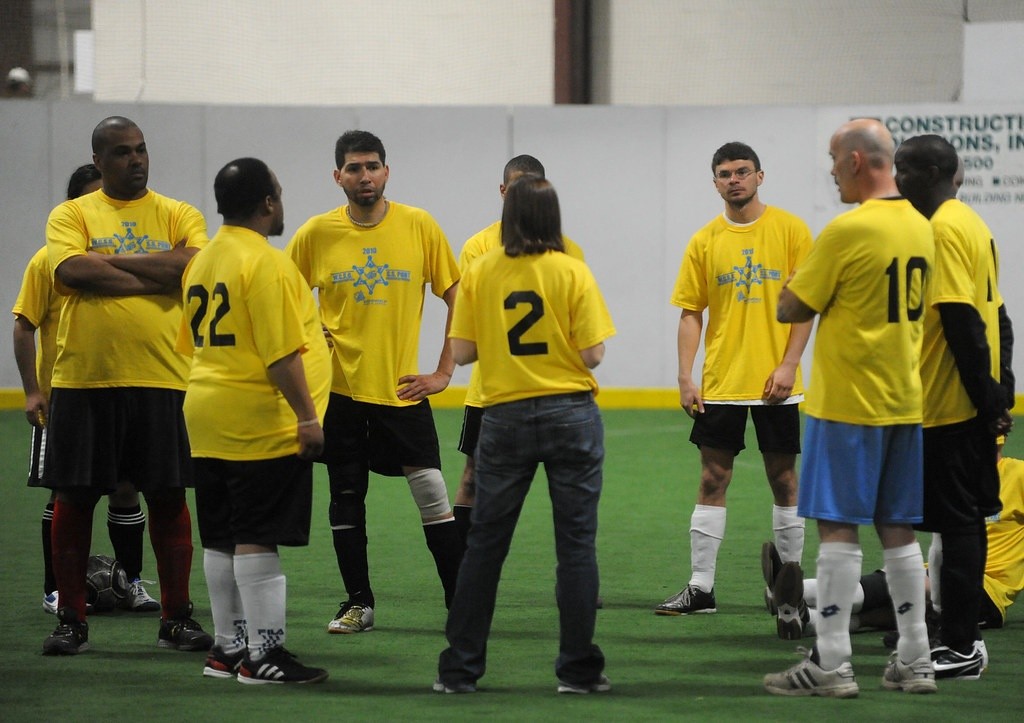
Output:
[761,542,783,616]
[431,678,457,693]
[204,644,246,677]
[129,577,160,611]
[764,646,859,697]
[328,588,374,633]
[43,605,89,653]
[557,672,611,694]
[930,644,984,680]
[236,637,328,685]
[654,583,717,615]
[157,602,213,650]
[882,651,938,691]
[42,590,59,614]
[773,560,810,639]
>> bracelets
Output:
[296,418,319,426]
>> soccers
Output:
[86,555,129,613]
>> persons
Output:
[454,152,585,546]
[38,113,212,657]
[760,119,936,695]
[8,161,163,616]
[281,128,458,634]
[435,172,614,697]
[657,140,816,614]
[180,156,333,686]
[889,132,1014,677]
[758,450,1024,637]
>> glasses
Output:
[714,167,758,182]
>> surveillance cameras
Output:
[7,68,30,87]
